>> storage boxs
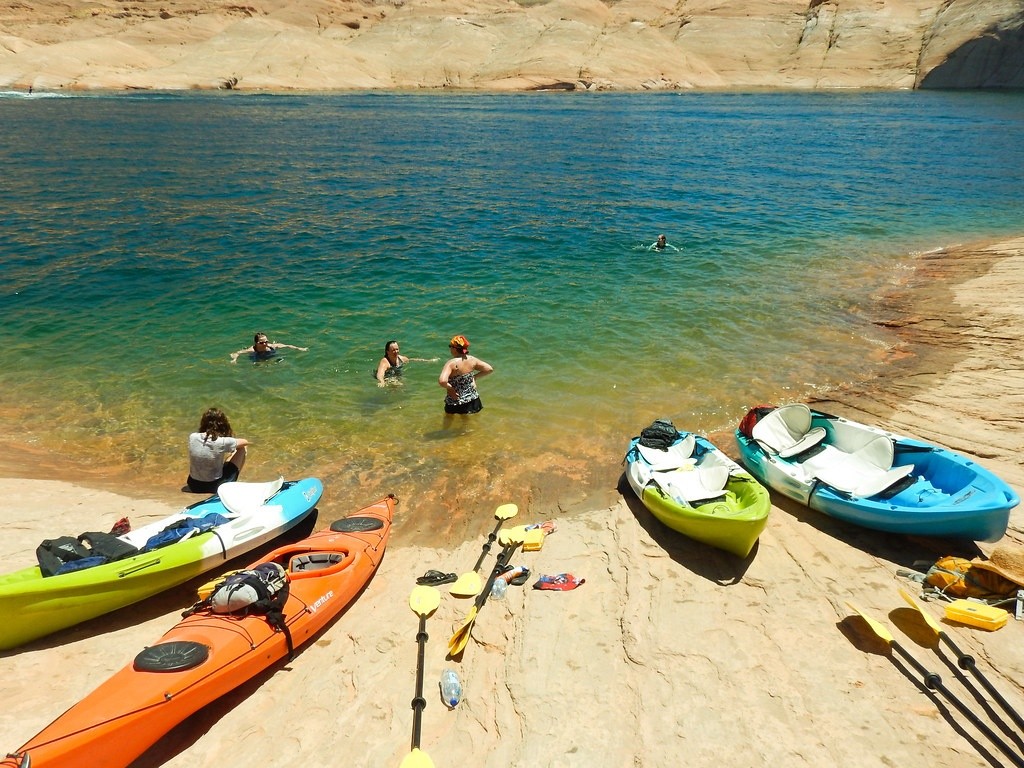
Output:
[945,598,1009,631]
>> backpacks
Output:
[618,418,683,466]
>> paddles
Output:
[402,585,440,768]
[447,525,526,658]
[896,585,1024,730]
[843,599,1024,768]
[448,503,520,597]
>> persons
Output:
[376,341,441,388]
[230,332,308,364]
[653,232,673,247]
[186,407,248,493]
[439,335,493,416]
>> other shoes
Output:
[108,516,132,538]
[505,564,532,586]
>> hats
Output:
[969,546,1024,588]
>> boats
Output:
[625,431,771,559]
[0,476,324,651]
[0,493,399,768]
[735,405,1021,543]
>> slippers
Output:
[416,568,458,587]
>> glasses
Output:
[448,345,453,348]
[257,340,269,345]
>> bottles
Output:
[440,668,463,707]
[495,566,529,584]
[492,577,507,600]
